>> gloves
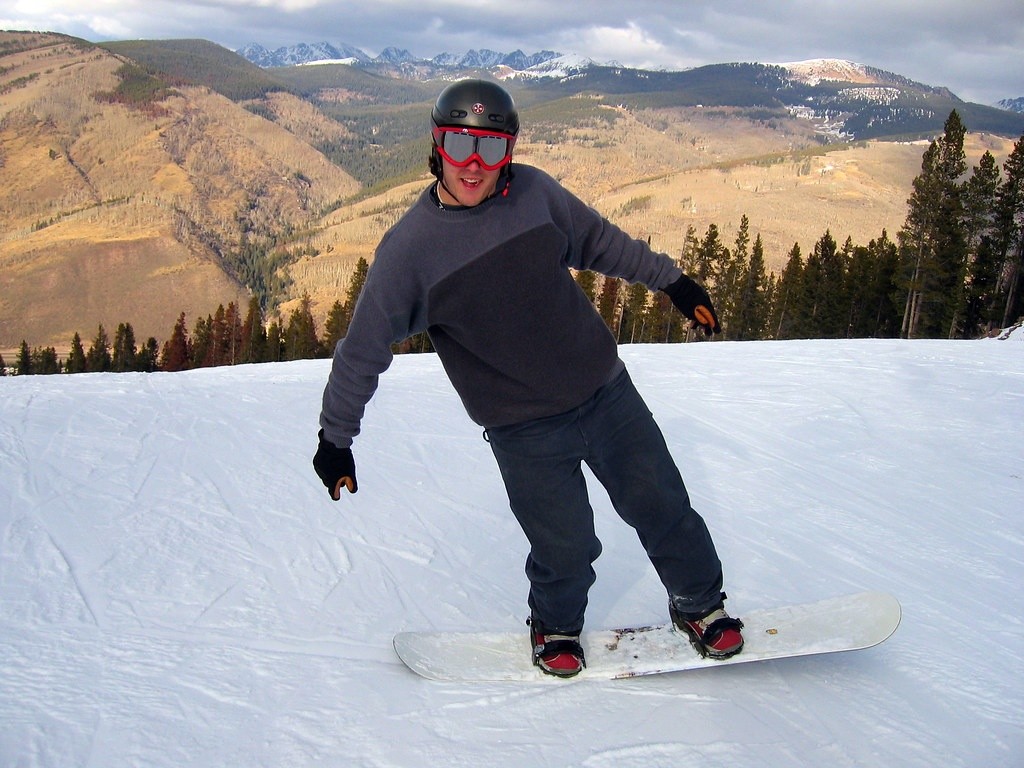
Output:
[312,428,358,501]
[661,273,721,337]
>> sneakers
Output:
[668,601,744,659]
[531,624,582,678]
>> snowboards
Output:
[390,588,905,686]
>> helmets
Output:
[431,78,520,153]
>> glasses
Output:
[429,110,519,177]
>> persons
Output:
[311,78,746,680]
